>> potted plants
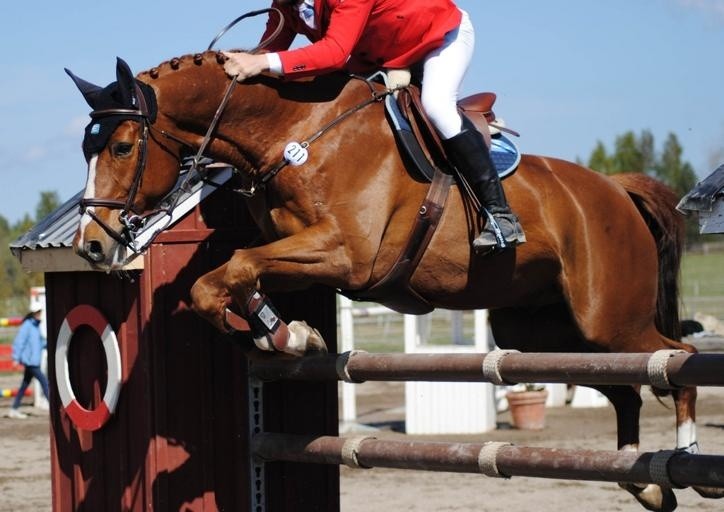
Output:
[506,384,549,430]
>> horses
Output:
[59,43,724,512]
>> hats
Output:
[29,303,42,313]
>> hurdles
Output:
[44,229,724,512]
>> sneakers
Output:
[8,409,28,419]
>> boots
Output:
[441,108,527,248]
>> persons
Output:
[215,1,527,256]
[5,304,50,418]
